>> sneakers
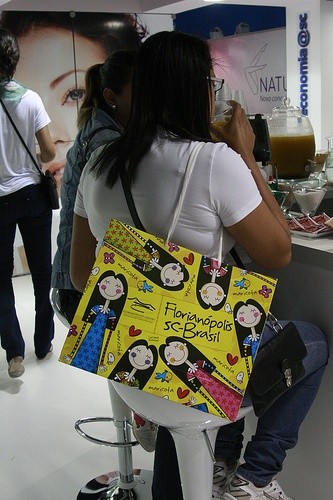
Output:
[222,471,296,500]
[212,461,242,499]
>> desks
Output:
[224,235,333,500]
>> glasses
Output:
[204,76,224,92]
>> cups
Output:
[291,189,327,217]
[271,190,289,208]
[209,101,234,142]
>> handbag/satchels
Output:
[249,322,306,402]
[40,169,59,209]
[57,142,279,422]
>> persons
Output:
[50,48,160,452]
[0,31,56,377]
[70,29,329,499]
[1,9,150,198]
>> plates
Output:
[291,230,332,237]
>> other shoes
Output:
[8,356,25,378]
[49,343,53,351]
[133,411,157,452]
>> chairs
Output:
[49,289,253,500]
